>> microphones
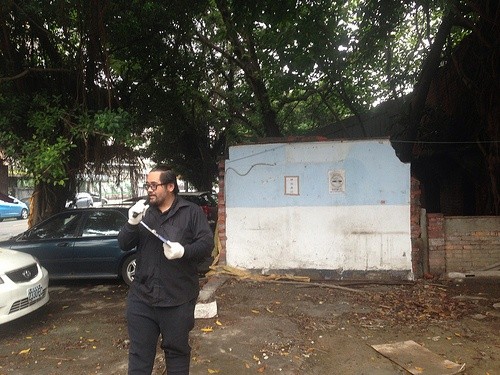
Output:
[133,196,150,218]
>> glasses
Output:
[143,182,167,191]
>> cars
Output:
[0,248,50,325]
[90,193,108,207]
[0,197,29,222]
[0,207,138,288]
[64,192,94,208]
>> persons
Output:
[116,165,215,375]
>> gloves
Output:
[162,240,185,261]
[127,199,150,225]
